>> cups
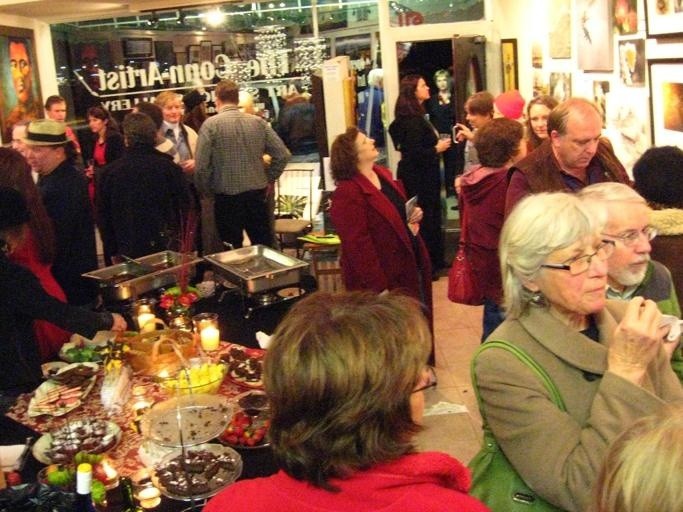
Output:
[440,134,451,140]
[180,154,191,164]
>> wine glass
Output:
[87,160,95,175]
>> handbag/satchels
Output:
[447,247,487,308]
[454,340,569,511]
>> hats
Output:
[20,118,72,146]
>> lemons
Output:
[165,363,226,394]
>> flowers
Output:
[159,284,202,310]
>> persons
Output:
[388,75,451,281]
[574,180,682,387]
[201,290,493,511]
[329,126,435,365]
[589,403,683,511]
[0,35,44,143]
[474,190,683,512]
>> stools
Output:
[300,241,344,292]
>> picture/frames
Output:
[645,56,683,150]
[63,35,224,124]
[641,1,683,40]
[501,36,518,95]
[0,23,46,147]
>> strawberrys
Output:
[219,412,271,448]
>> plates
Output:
[31,419,124,466]
[36,464,121,496]
[137,395,232,448]
[151,443,245,504]
[214,392,270,450]
[27,298,270,395]
[27,362,99,417]
[277,288,305,298]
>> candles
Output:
[136,313,158,330]
[196,326,222,350]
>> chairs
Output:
[270,165,316,260]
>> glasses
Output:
[602,227,657,246]
[540,239,615,276]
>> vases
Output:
[167,310,193,335]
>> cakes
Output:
[44,419,116,463]
[155,450,236,497]
[237,393,267,409]
[217,347,265,386]
